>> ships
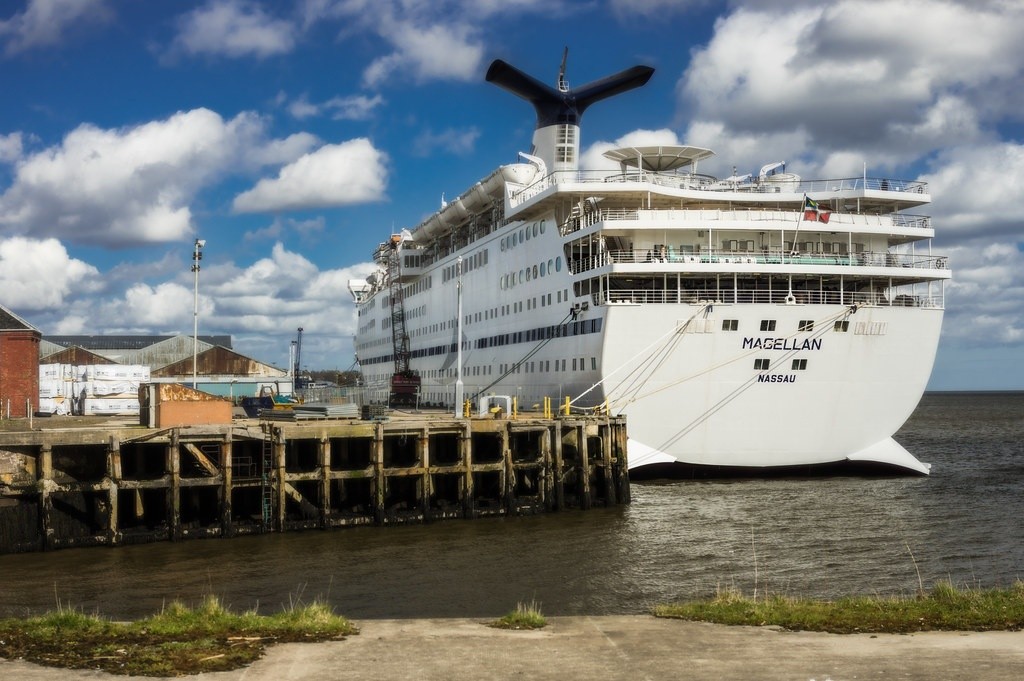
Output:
[346,47,952,480]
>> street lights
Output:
[454,256,464,419]
[191,238,206,389]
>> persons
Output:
[660,244,665,263]
[881,179,888,190]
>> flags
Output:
[803,196,832,223]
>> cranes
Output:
[388,236,421,408]
